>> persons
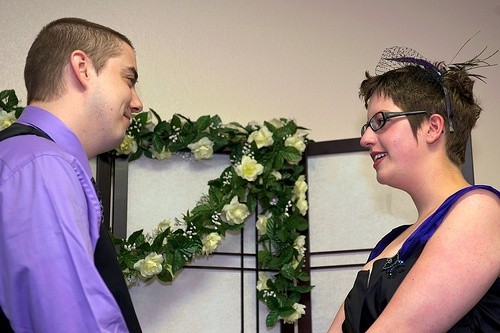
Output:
[0,17,143,333]
[326,45,500,333]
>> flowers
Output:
[0,89,316,330]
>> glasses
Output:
[361,110,444,137]
